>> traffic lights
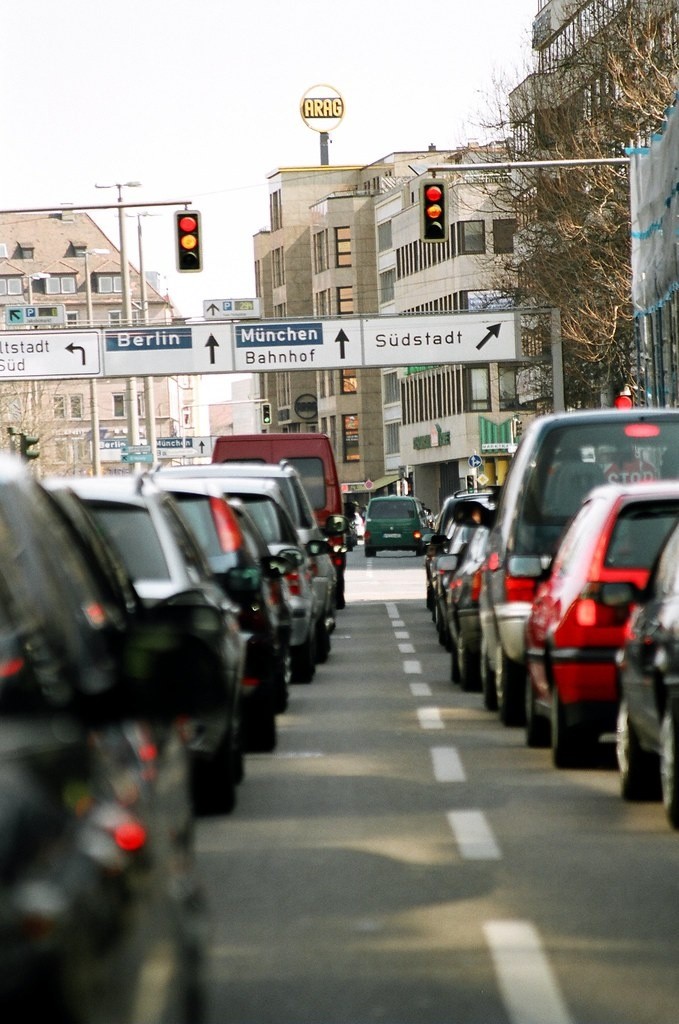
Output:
[174,210,203,273]
[261,403,272,425]
[420,179,450,245]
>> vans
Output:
[447,407,678,726]
[364,496,430,558]
[211,433,359,610]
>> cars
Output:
[130,462,336,747]
[592,514,679,827]
[498,481,679,771]
[421,486,497,691]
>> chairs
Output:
[541,462,605,515]
[614,512,669,566]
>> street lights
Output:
[113,208,160,469]
[94,181,144,475]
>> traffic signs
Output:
[103,321,237,376]
[365,315,519,364]
[235,319,363,372]
[0,330,103,381]
[155,436,218,459]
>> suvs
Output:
[0,460,245,1024]
[41,470,255,817]
